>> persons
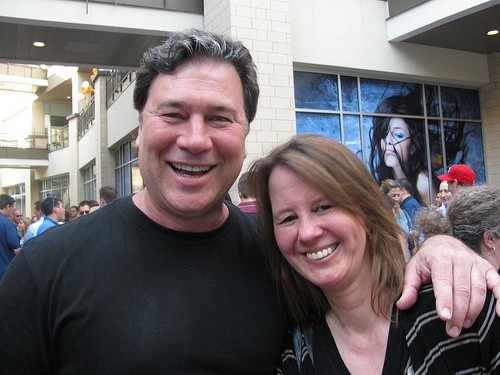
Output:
[0,26,500,375]
[356,81,477,207]
[377,164,500,273]
[0,186,118,280]
[236,171,261,213]
[246,133,500,375]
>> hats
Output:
[437,164,476,184]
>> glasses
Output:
[14,214,22,218]
[79,211,90,216]
